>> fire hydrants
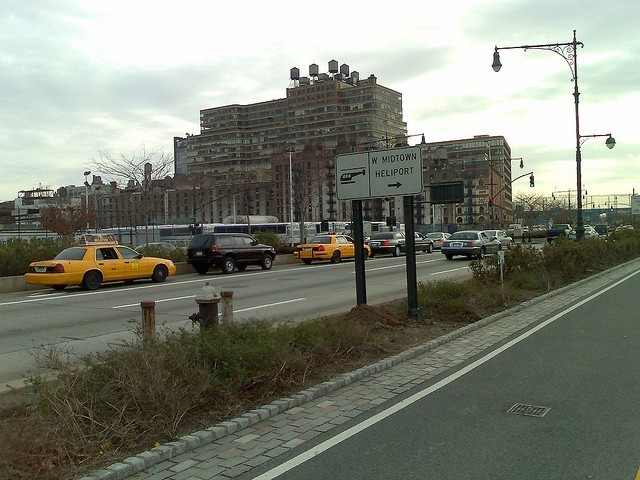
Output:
[189,283,222,332]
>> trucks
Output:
[507,224,521,236]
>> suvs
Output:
[187,233,276,273]
[594,225,608,236]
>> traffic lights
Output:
[520,161,524,169]
[530,176,534,187]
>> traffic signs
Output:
[631,196,640,214]
[336,146,423,199]
[430,182,464,204]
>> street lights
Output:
[287,146,296,247]
[554,195,584,200]
[350,133,426,232]
[84,171,91,230]
[552,190,588,227]
[492,29,616,240]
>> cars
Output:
[484,230,512,247]
[524,226,528,233]
[414,232,433,253]
[425,233,447,250]
[444,233,451,238]
[24,245,176,291]
[547,224,572,244]
[294,235,372,264]
[441,231,501,260]
[615,226,627,236]
[626,225,634,235]
[365,232,406,258]
[569,225,599,240]
